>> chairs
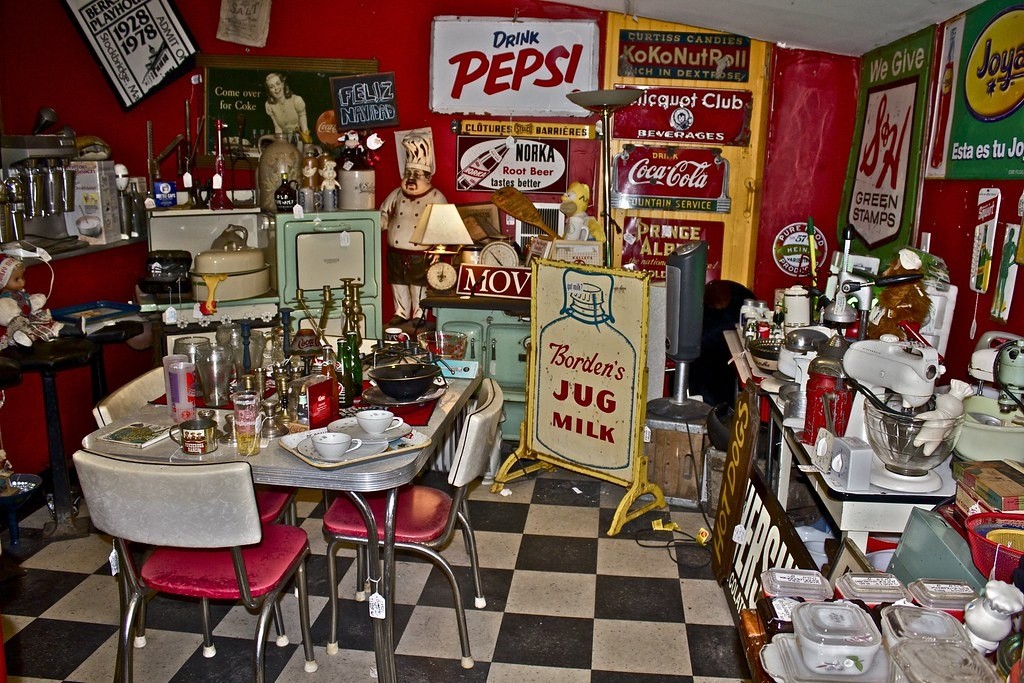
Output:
[76,328,504,682]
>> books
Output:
[100,421,180,449]
[952,459,1024,521]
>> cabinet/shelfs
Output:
[421,295,532,443]
[0,208,381,528]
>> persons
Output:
[991,228,1017,318]
[265,74,307,142]
[380,135,448,328]
[0,253,86,349]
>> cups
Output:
[564,217,589,241]
[311,432,362,458]
[163,323,302,456]
[356,409,404,434]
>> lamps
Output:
[565,89,644,267]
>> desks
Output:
[80,356,484,683]
[725,327,958,553]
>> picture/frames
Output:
[194,54,380,171]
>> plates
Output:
[360,386,446,406]
[327,416,412,445]
[297,436,389,463]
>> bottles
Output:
[257,131,377,213]
[119,182,144,238]
[772,304,784,333]
[334,339,353,408]
[320,345,339,416]
[739,299,755,330]
[754,300,770,316]
[346,331,363,397]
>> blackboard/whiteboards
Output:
[328,71,401,133]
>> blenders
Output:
[752,216,1024,505]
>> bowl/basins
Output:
[862,391,962,477]
[748,338,785,375]
[755,567,1024,683]
[955,397,1024,463]
[416,330,468,360]
[75,215,101,236]
[367,363,442,402]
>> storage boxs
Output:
[64,161,123,245]
[951,461,1024,525]
[637,420,708,509]
